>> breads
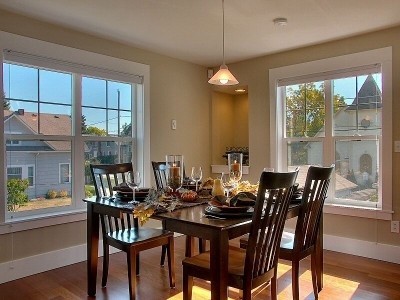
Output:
[181,190,197,201]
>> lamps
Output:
[207,0,240,86]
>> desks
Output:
[81,185,329,300]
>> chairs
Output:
[238,164,337,300]
[151,159,207,269]
[90,160,177,300]
[183,164,301,300]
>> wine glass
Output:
[166,153,274,203]
[126,171,140,204]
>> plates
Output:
[204,203,254,216]
[114,190,150,200]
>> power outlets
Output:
[390,221,400,233]
